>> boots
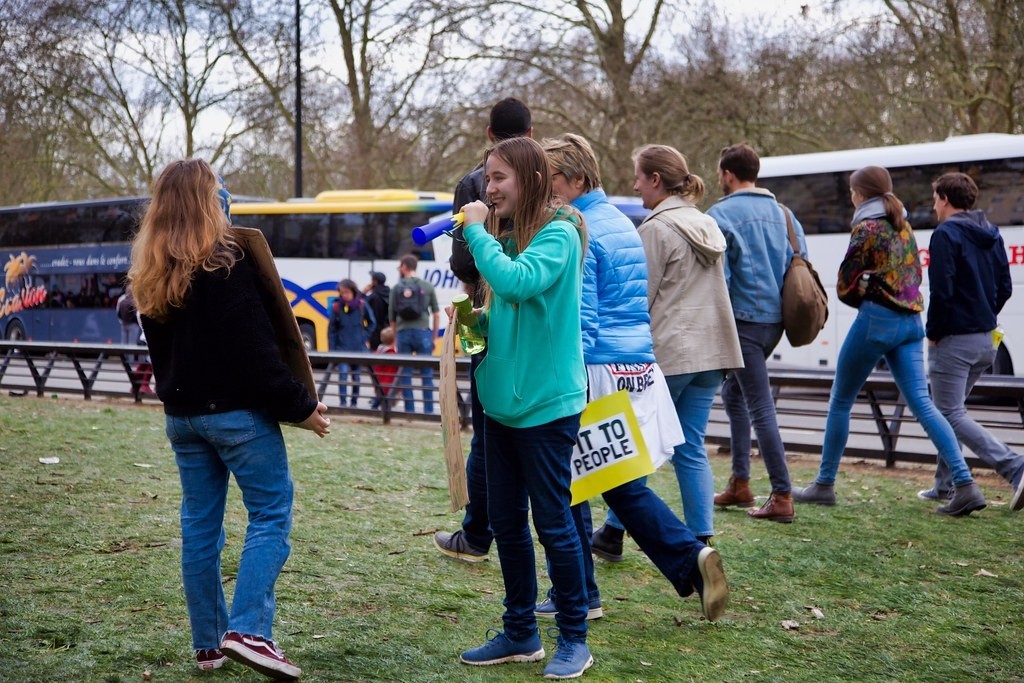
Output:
[130,360,156,395]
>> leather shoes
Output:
[748,490,794,524]
[712,475,755,508]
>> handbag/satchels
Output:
[782,255,829,349]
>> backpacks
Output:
[393,280,426,321]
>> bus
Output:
[746,133,1024,405]
[0,194,276,362]
[228,189,652,371]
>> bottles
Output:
[451,293,486,355]
[859,274,870,290]
[992,322,1005,351]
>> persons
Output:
[430,99,594,575]
[130,160,331,680]
[361,271,390,352]
[588,144,745,562]
[703,144,808,522]
[790,167,988,514]
[372,328,402,410]
[915,172,1024,512]
[458,138,594,680]
[329,279,375,407]
[388,256,440,414]
[115,287,156,395]
[536,132,730,621]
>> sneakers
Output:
[544,624,594,678]
[221,629,301,680]
[1009,465,1024,512]
[196,645,228,671]
[589,522,624,562]
[459,623,546,666]
[918,481,951,500]
[533,596,603,620]
[690,543,730,623]
[434,529,489,565]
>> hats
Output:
[370,270,386,286]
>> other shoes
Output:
[793,482,836,505]
[936,482,987,518]
